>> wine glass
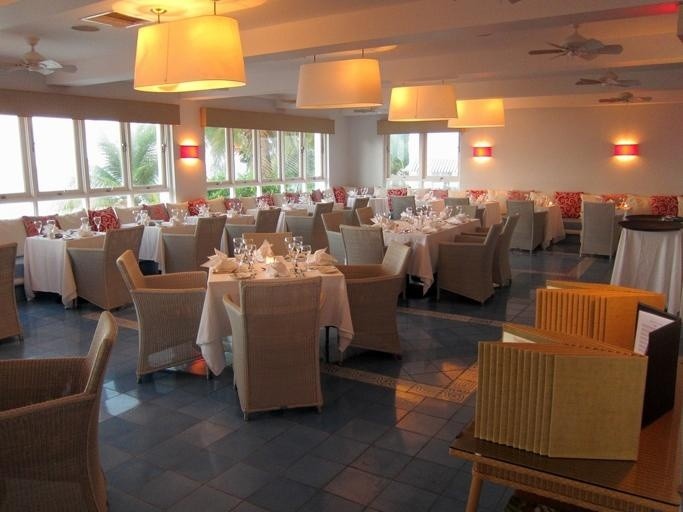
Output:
[383,190,463,225]
[33,184,370,237]
[233,236,312,277]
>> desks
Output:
[448,357,683,512]
[609,213,682,317]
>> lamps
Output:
[387,80,458,122]
[613,144,639,156]
[133,2,245,93]
[448,98,505,130]
[471,146,492,157]
[294,47,384,110]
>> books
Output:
[634,301,681,429]
[535,279,668,348]
[474,323,650,463]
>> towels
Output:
[43,222,94,237]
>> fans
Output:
[1,35,78,75]
[528,23,623,61]
[178,144,201,159]
[598,91,652,105]
[576,69,641,86]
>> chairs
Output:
[0,308,118,512]
[223,275,324,422]
[0,241,24,340]
[117,248,210,385]
[565,190,683,256]
